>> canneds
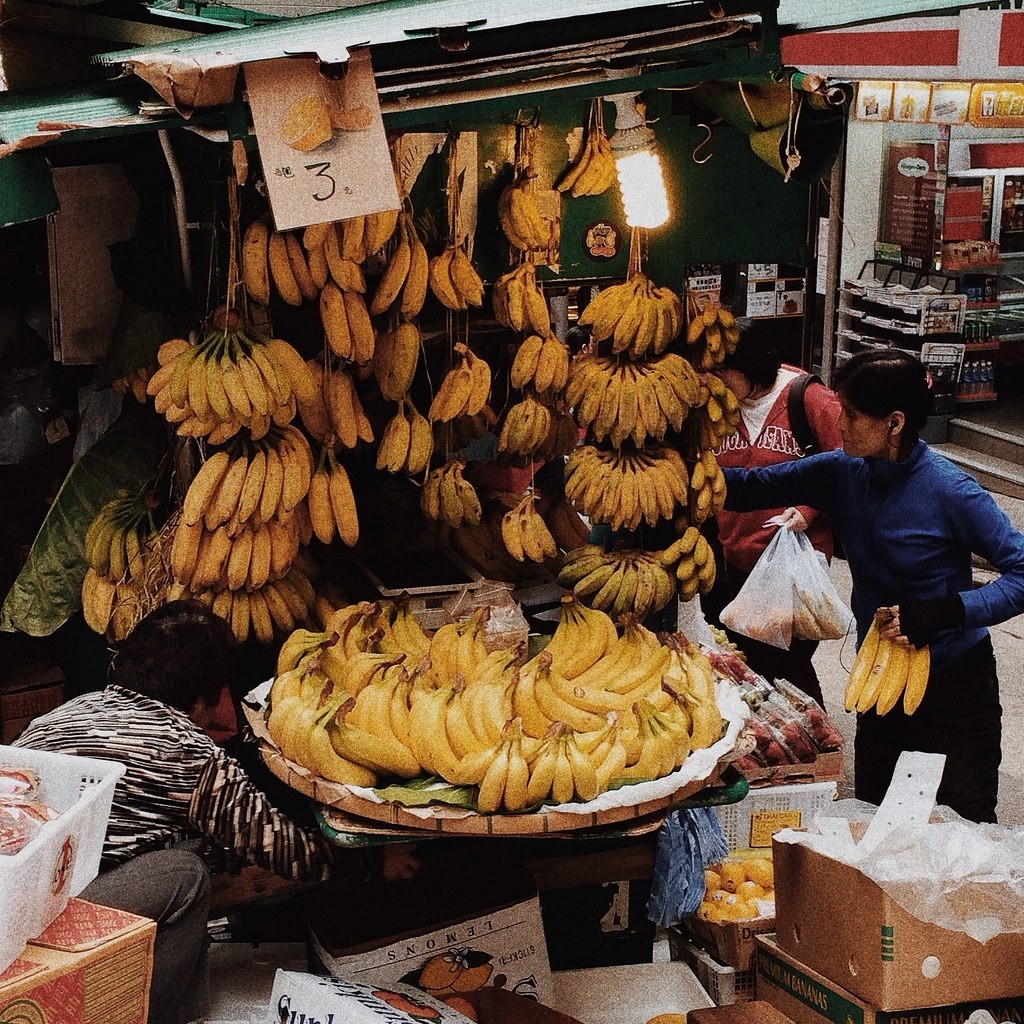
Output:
[963,322,992,340]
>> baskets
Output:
[0,745,126,975]
[707,781,839,862]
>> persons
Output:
[8,598,422,1024]
[698,321,841,715]
[722,350,1024,824]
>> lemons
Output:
[690,857,776,925]
[278,92,333,152]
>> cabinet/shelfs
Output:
[834,258,1024,413]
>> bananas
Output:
[78,129,738,643]
[844,609,929,717]
[264,590,723,814]
[789,581,850,641]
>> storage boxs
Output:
[0,899,158,1024]
[670,928,761,1007]
[266,968,477,1024]
[754,933,1024,1024]
[771,814,1024,1012]
[0,745,126,976]
[303,856,555,1009]
[677,916,777,972]
[705,781,838,856]
[712,748,845,791]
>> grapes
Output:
[707,624,748,663]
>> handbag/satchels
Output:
[716,524,856,652]
